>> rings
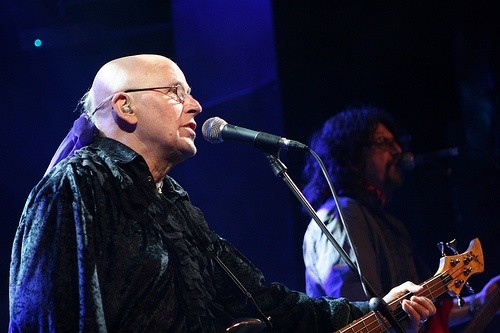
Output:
[420,318,428,323]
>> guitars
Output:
[224,237,486,333]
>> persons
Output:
[7,54,437,333]
[301,105,500,333]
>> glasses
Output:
[92,83,191,116]
